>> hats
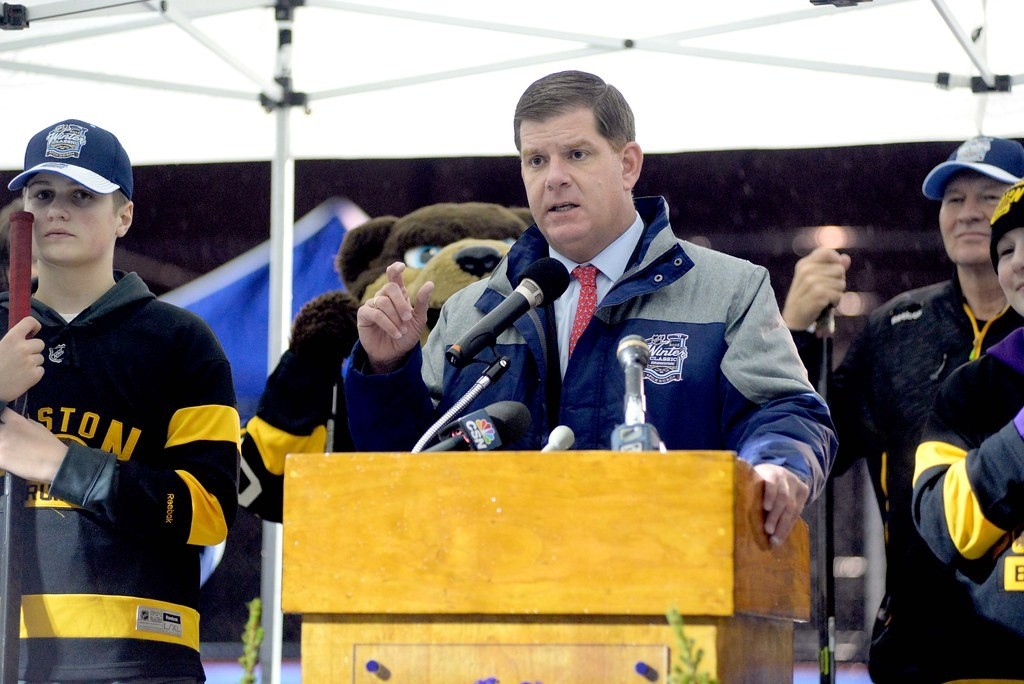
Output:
[920,138,1024,196]
[987,180,1023,273]
[8,120,136,199]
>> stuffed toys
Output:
[236,202,545,543]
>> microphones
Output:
[422,401,532,451]
[616,334,651,424]
[540,425,575,451]
[445,257,570,369]
[816,303,835,338]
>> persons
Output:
[0,197,44,293]
[338,69,839,550]
[912,176,1024,684]
[0,117,242,684]
[783,135,1024,684]
